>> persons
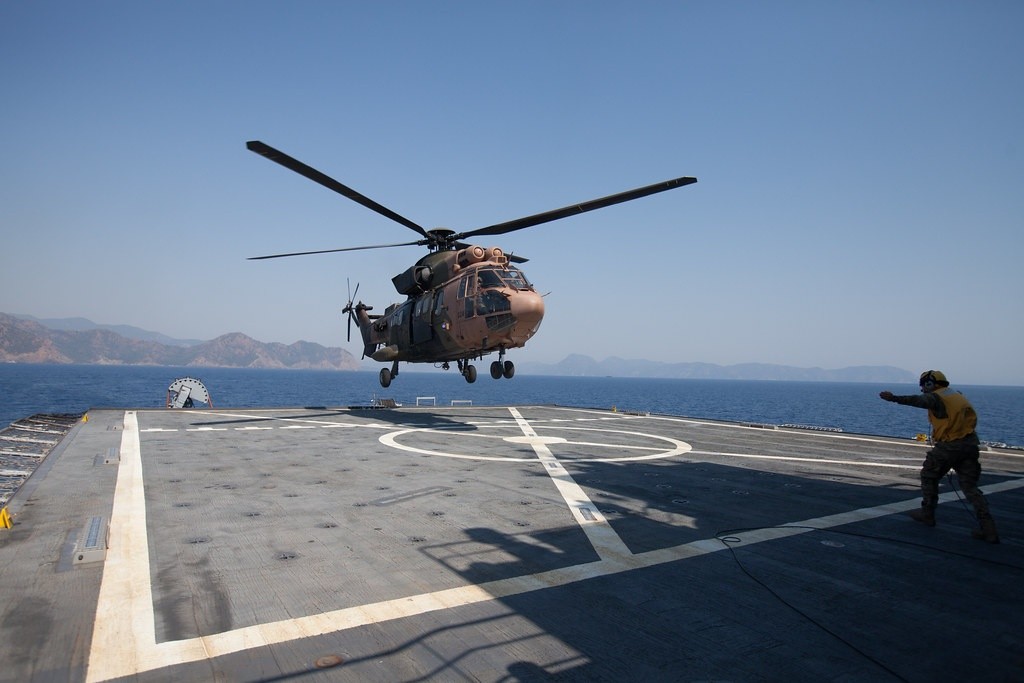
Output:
[880,370,1000,544]
[468,276,490,312]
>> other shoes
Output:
[972,523,1000,544]
[910,509,935,527]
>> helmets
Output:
[919,370,949,393]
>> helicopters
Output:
[243,140,698,389]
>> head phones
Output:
[925,370,935,390]
